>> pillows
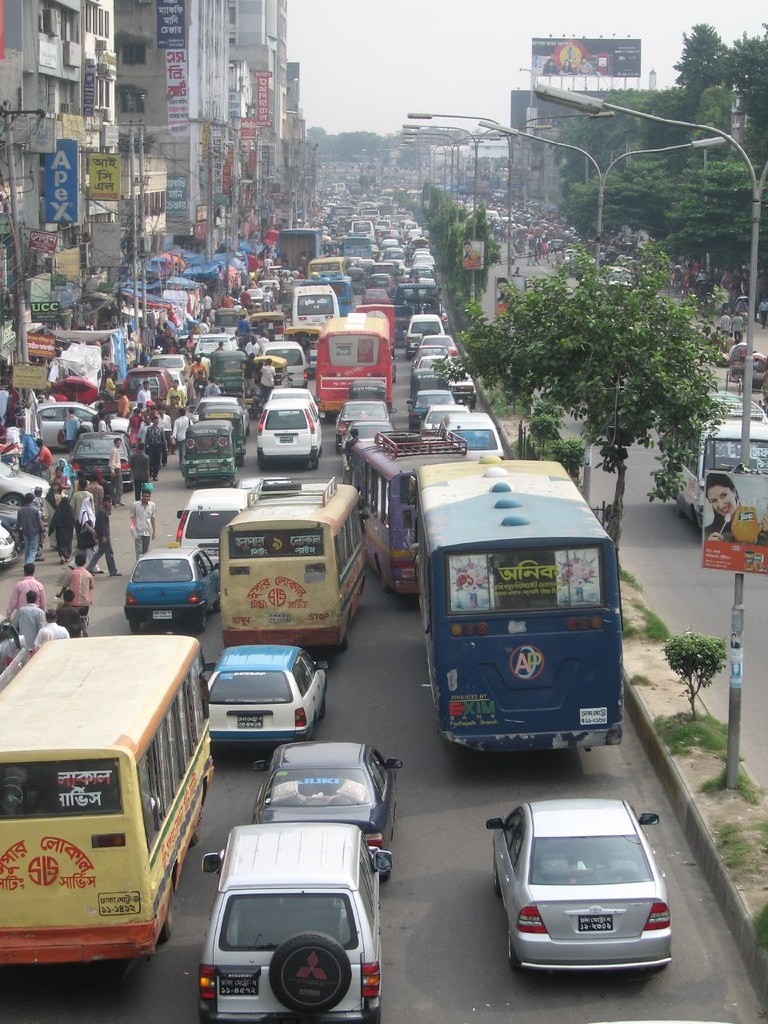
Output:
[272,781,299,804]
[337,778,366,803]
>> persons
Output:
[454,192,542,255]
[259,209,337,285]
[462,242,482,267]
[672,253,768,348]
[496,278,511,315]
[705,473,768,545]
[23,356,221,481]
[153,282,276,410]
[540,59,593,76]
[527,210,674,297]
[730,633,740,649]
[8,438,157,576]
[6,553,94,650]
[344,427,362,484]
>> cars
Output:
[0,280,280,566]
[326,198,439,304]
[335,399,392,464]
[483,796,675,977]
[208,643,405,866]
[403,312,506,464]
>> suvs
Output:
[199,822,392,1024]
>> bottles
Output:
[745,549,754,569]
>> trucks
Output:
[278,227,322,270]
[393,283,448,335]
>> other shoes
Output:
[86,569,96,576]
[111,572,123,576]
[117,501,125,506]
[153,476,158,480]
[96,570,105,574]
[66,558,70,561]
[60,558,65,564]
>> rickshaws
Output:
[725,342,749,395]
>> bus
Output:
[290,221,374,328]
[0,633,217,966]
[218,423,626,752]
[670,390,768,535]
[316,313,395,415]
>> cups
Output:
[732,498,759,545]
[754,553,768,571]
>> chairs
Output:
[538,859,572,879]
[608,859,638,876]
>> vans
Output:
[256,387,325,471]
[262,339,311,390]
[122,548,218,632]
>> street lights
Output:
[400,110,619,307]
[531,82,768,791]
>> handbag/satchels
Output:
[77,510,97,549]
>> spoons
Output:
[719,512,731,534]
